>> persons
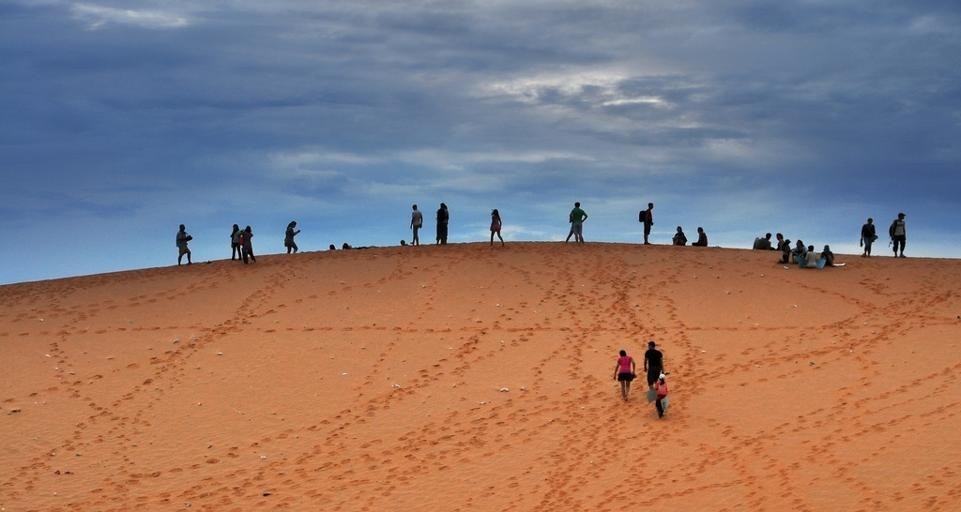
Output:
[754,232,834,268]
[566,222,573,242]
[241,225,256,264]
[286,221,300,253]
[490,209,504,246]
[231,224,241,260]
[644,203,653,244]
[570,202,588,242]
[862,219,875,256]
[614,341,667,418]
[891,213,906,257]
[673,226,687,246]
[401,202,448,246]
[176,224,191,265]
[692,227,707,247]
